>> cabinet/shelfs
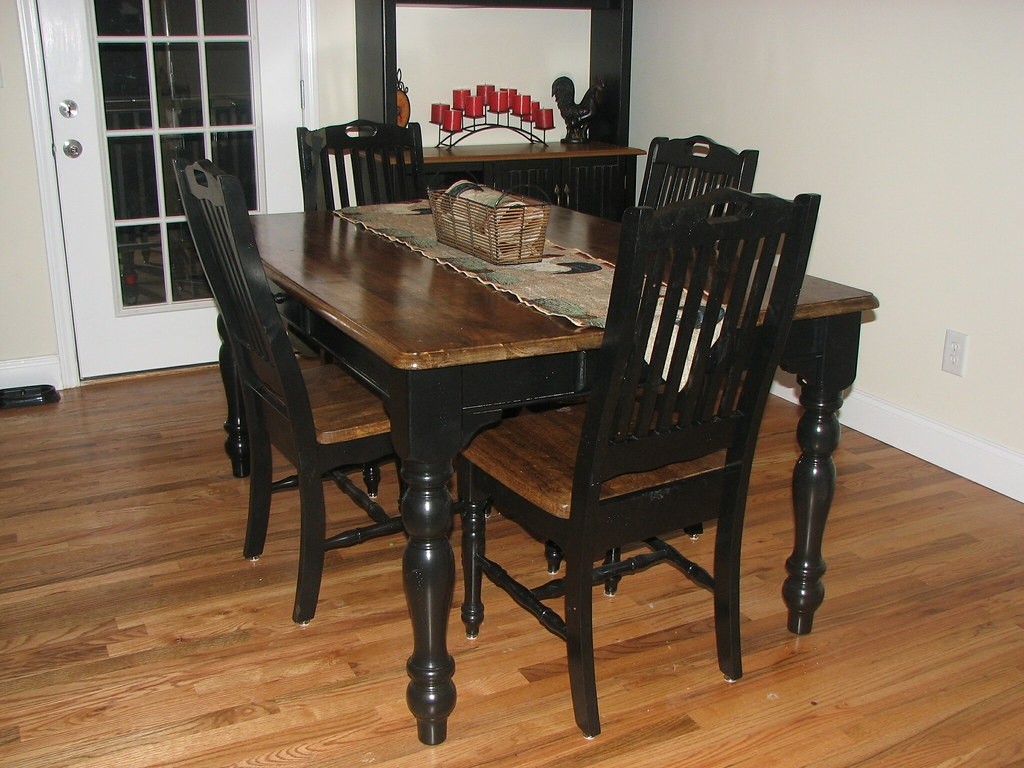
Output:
[358,139,647,225]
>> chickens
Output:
[551,77,608,144]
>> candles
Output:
[464,96,484,117]
[512,94,531,116]
[501,88,517,109]
[535,109,554,128]
[443,110,461,132]
[490,90,507,113]
[523,101,541,122]
[431,103,450,125]
[477,85,495,105]
[453,89,471,110]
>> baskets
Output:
[425,164,552,266]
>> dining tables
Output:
[247,192,879,745]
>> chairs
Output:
[453,186,822,740]
[170,157,407,625]
[636,135,759,542]
[295,119,427,367]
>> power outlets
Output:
[940,329,969,377]
